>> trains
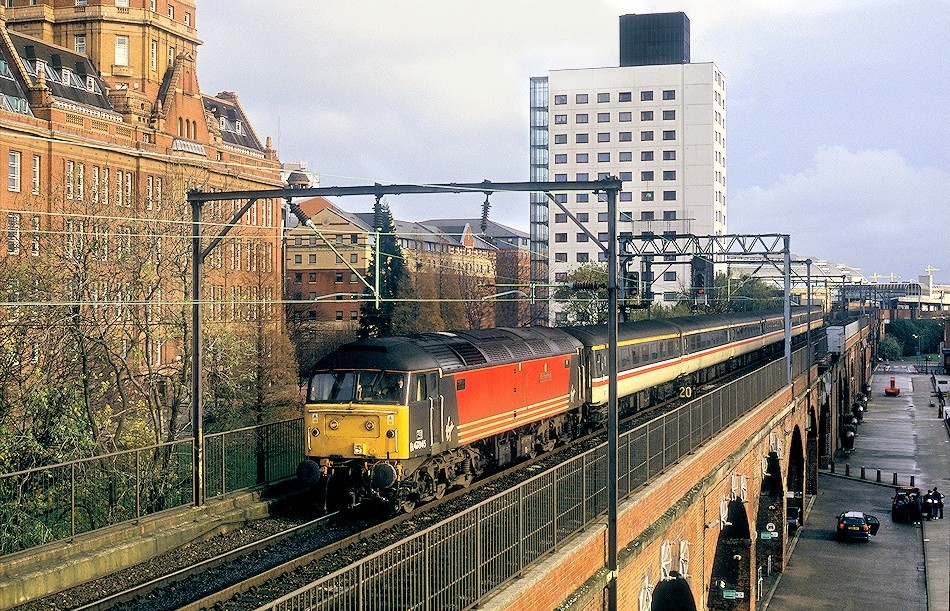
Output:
[294,305,825,518]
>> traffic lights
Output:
[689,255,714,295]
[627,272,639,295]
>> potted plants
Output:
[841,381,872,448]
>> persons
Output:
[932,486,945,520]
[902,491,916,526]
[923,489,934,521]
[389,375,404,403]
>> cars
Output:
[835,510,880,543]
[890,485,924,525]
